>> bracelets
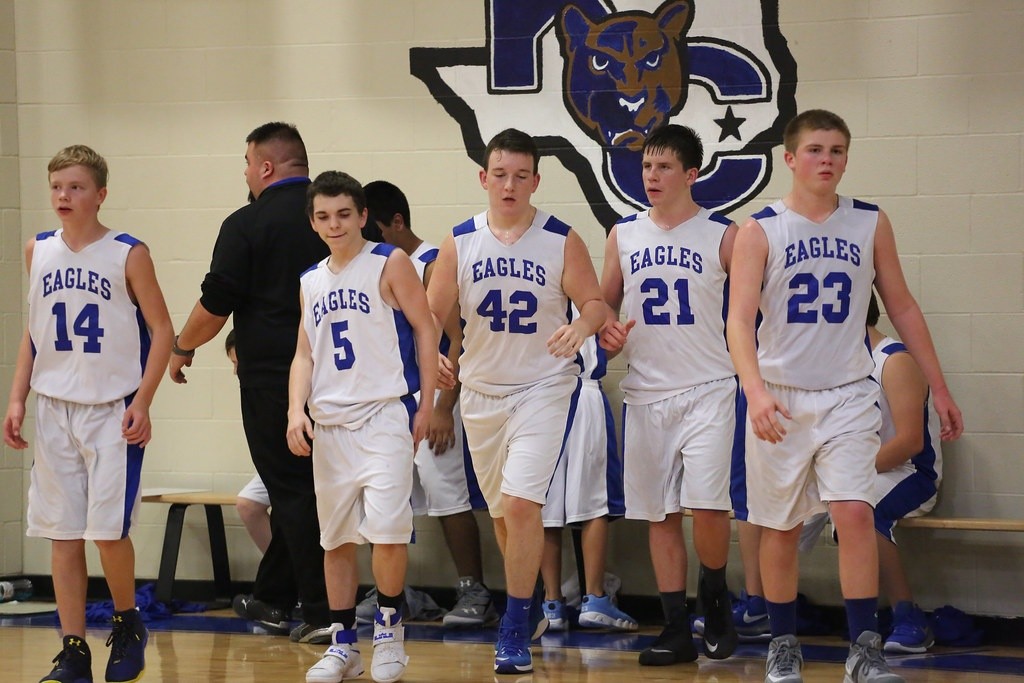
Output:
[172,336,195,356]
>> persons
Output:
[726,110,964,683]
[596,125,739,667]
[425,128,607,675]
[3,145,175,683]
[866,288,943,652]
[169,123,387,645]
[285,171,439,683]
[225,181,820,638]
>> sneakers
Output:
[579,595,638,630]
[443,577,499,627]
[289,621,331,643]
[638,615,698,665]
[233,594,290,628]
[702,589,735,659]
[765,634,803,683]
[306,620,365,683]
[541,597,568,630]
[370,608,409,683]
[494,601,550,656]
[884,600,934,652]
[494,617,532,673]
[39,637,93,683]
[843,631,906,683]
[356,584,422,624]
[105,608,149,683]
[694,596,771,635]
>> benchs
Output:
[141,492,1024,618]
